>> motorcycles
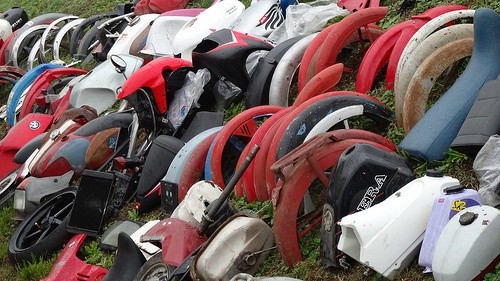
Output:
[0,54,150,268]
